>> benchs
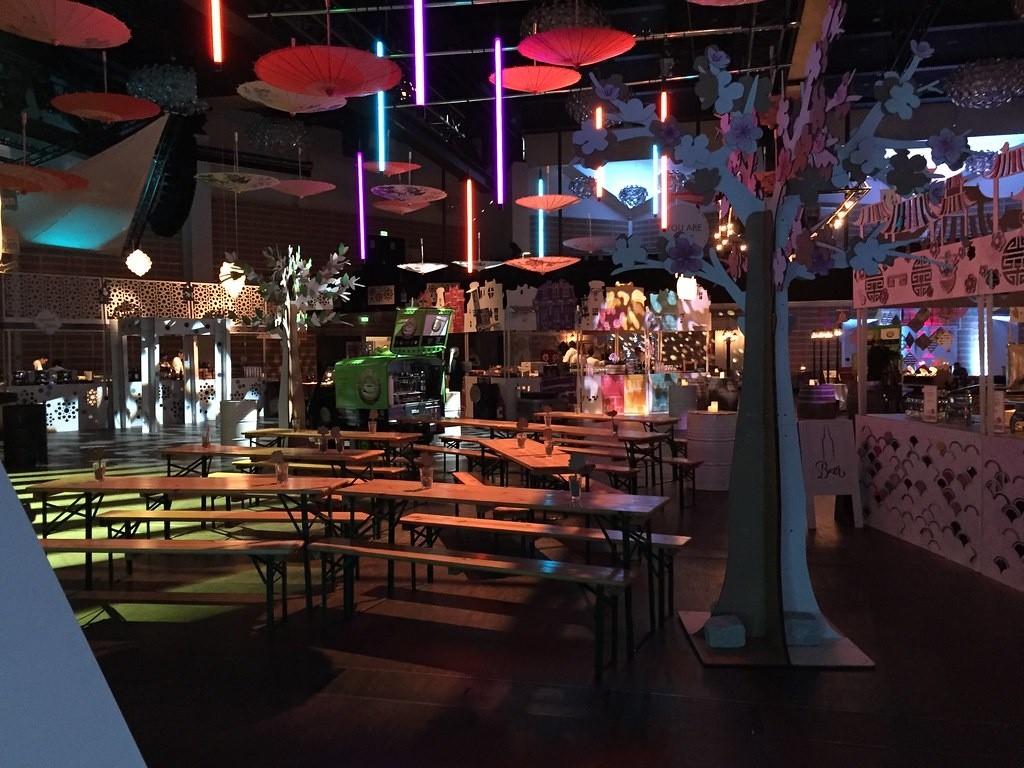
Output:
[37,430,704,684]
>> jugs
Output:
[85,371,92,381]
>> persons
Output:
[158,349,214,380]
[562,341,654,374]
[32,354,64,370]
[866,346,903,412]
[446,344,482,393]
[953,363,970,387]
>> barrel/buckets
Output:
[797,384,836,420]
[220,400,258,461]
[1,404,47,470]
[669,385,698,431]
[685,408,738,492]
[473,383,498,420]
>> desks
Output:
[163,444,387,536]
[470,436,592,521]
[534,411,681,485]
[337,480,671,665]
[239,430,423,479]
[435,418,666,489]
[26,473,346,637]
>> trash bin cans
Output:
[2,403,49,467]
[220,400,259,459]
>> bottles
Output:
[903,398,950,421]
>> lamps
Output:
[219,131,246,299]
[126,240,152,279]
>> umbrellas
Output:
[235,0,401,116]
[0,113,89,195]
[489,0,763,95]
[50,50,161,124]
[196,132,610,275]
[1,0,131,49]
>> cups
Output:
[544,414,551,427]
[318,438,328,453]
[516,433,526,449]
[419,468,434,488]
[574,407,580,414]
[275,463,288,483]
[335,437,344,454]
[201,435,211,449]
[544,440,554,457]
[292,419,301,433]
[368,421,377,434]
[568,475,582,499]
[92,461,107,483]
